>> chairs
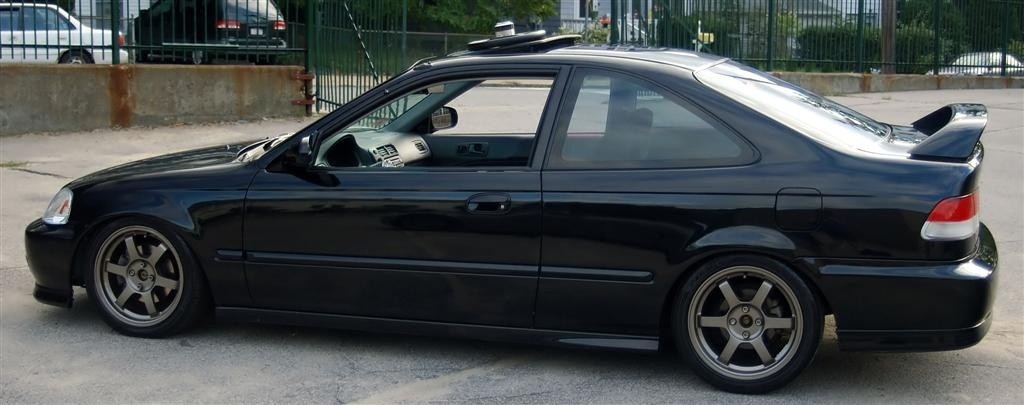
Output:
[586,107,654,162]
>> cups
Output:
[495,22,515,37]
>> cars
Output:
[0,2,130,65]
[924,51,1024,77]
[22,21,1000,397]
[126,0,289,67]
